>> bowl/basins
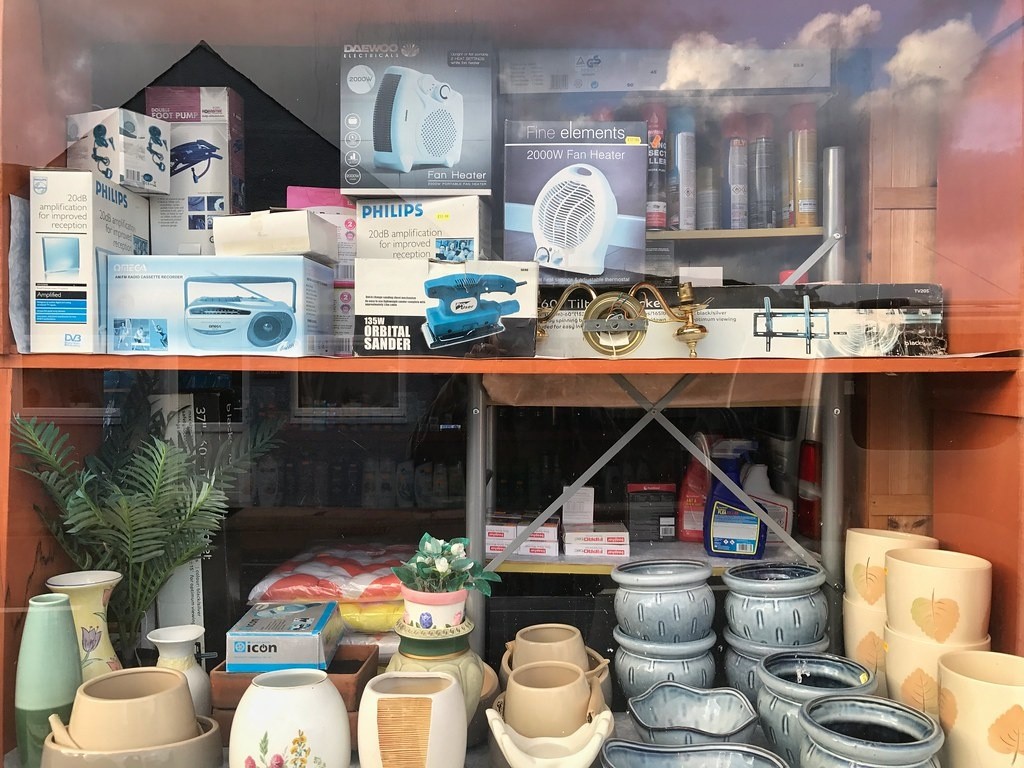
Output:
[626,680,760,747]
[467,660,500,747]
[599,737,790,768]
[40,667,221,768]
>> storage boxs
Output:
[30,36,946,751]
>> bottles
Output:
[228,668,351,768]
[44,570,125,683]
[146,625,211,718]
[14,594,84,768]
[357,672,467,768]
[594,100,818,232]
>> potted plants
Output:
[6,383,300,670]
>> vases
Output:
[13,529,1024,768]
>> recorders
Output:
[183,276,297,353]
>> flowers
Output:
[390,531,503,600]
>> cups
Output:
[502,661,589,739]
[842,529,1024,768]
[511,625,590,673]
